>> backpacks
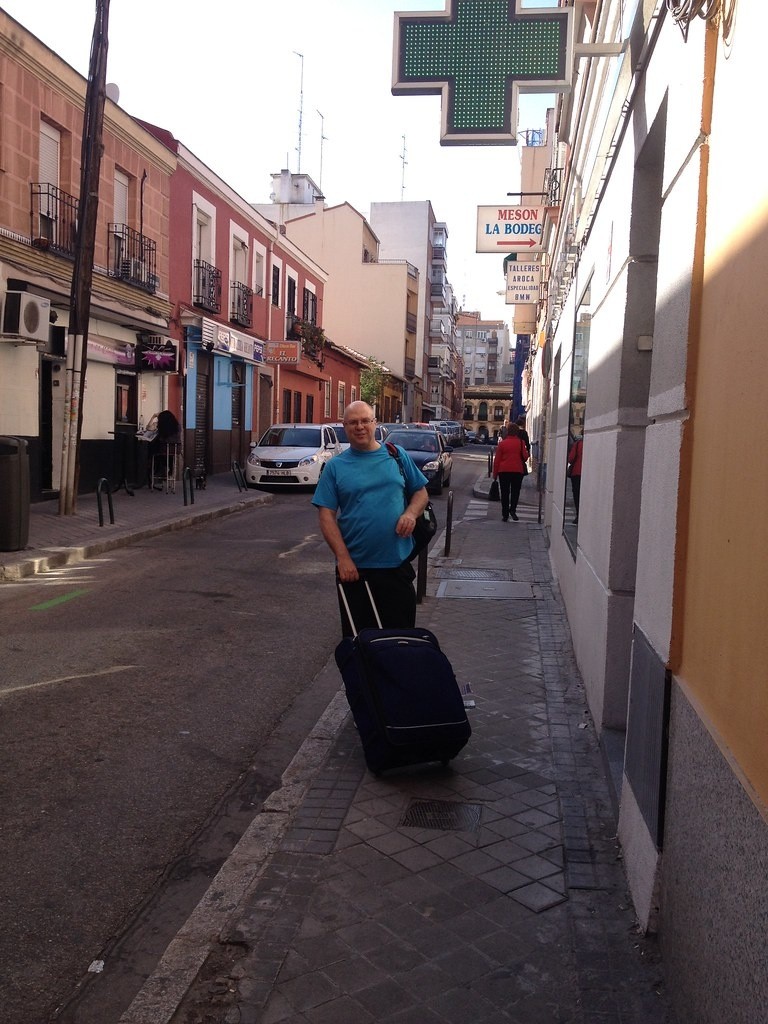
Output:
[384,441,438,560]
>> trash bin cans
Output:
[1,434,31,552]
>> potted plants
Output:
[291,317,325,355]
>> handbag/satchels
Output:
[488,480,499,501]
[566,464,573,477]
[520,439,529,476]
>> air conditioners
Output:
[0,291,50,343]
[146,334,179,376]
[119,256,148,286]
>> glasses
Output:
[342,418,376,426]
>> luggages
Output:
[335,574,472,779]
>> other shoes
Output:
[502,509,519,522]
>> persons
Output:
[514,418,530,458]
[310,401,429,731]
[497,418,510,441]
[493,422,530,523]
[568,428,584,525]
[418,436,436,452]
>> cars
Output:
[372,425,388,443]
[384,422,415,435]
[326,424,351,451]
[245,422,343,492]
[381,428,454,496]
[413,418,480,447]
